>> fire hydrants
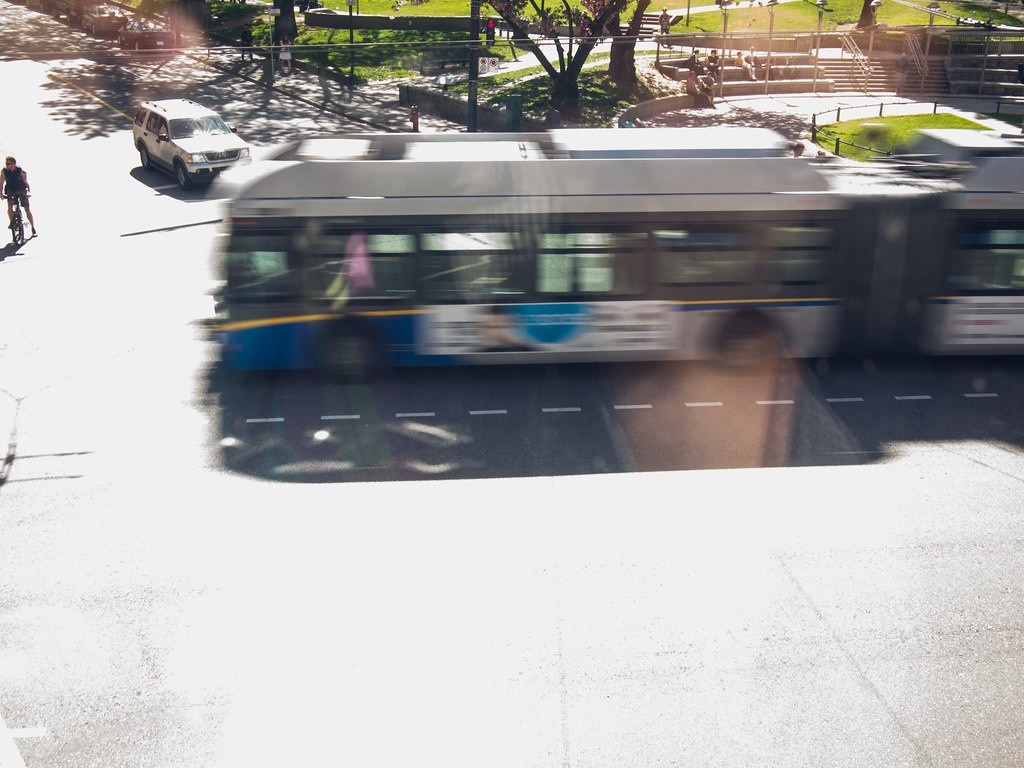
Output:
[410,103,419,132]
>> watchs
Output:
[686,50,705,75]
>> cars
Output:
[26,0,106,24]
[117,17,176,52]
[81,4,129,36]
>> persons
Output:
[688,70,716,109]
[241,24,254,61]
[544,100,563,129]
[706,50,720,79]
[894,52,908,94]
[734,46,761,81]
[658,7,672,47]
[278,33,291,71]
[0,156,39,238]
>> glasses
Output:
[6,163,12,165]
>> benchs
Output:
[433,57,470,70]
[620,14,662,40]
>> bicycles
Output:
[4,190,24,247]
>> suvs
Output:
[132,97,249,193]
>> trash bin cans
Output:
[620,121,638,128]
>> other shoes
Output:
[32,231,38,237]
[8,222,16,229]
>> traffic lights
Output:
[486,19,496,45]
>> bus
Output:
[199,127,1023,377]
